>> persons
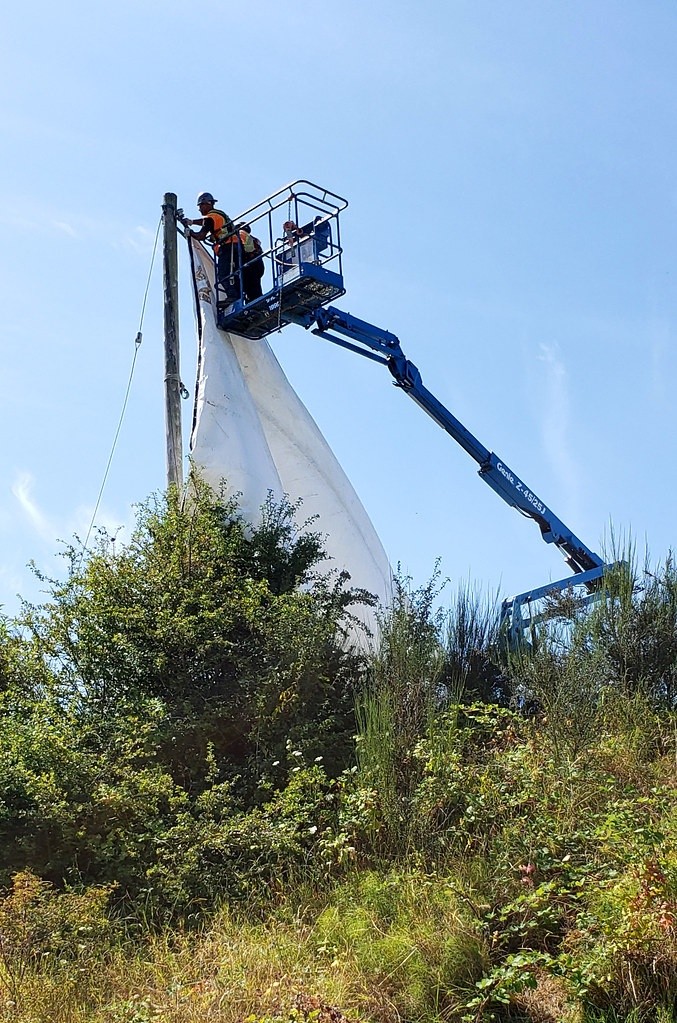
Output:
[235,222,270,312]
[184,192,244,308]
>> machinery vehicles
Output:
[200,178,676,720]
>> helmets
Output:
[235,222,251,234]
[197,192,218,206]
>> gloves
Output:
[181,218,193,226]
[183,228,194,237]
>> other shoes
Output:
[216,297,239,309]
[250,304,271,316]
